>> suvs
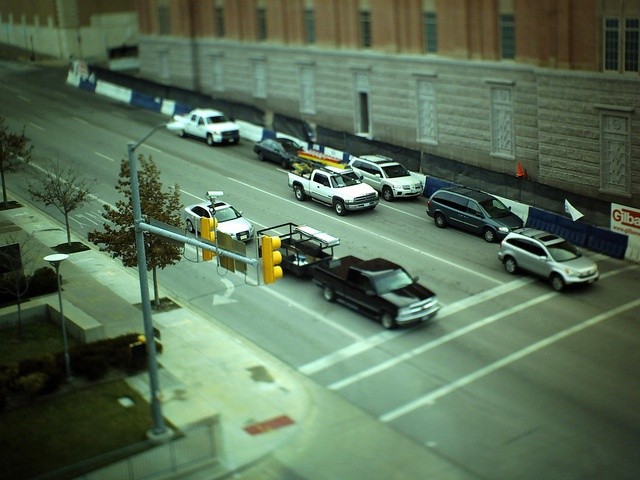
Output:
[345,154,423,201]
[498,228,599,291]
[426,187,524,243]
[173,109,239,145]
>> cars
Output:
[254,138,304,168]
[184,201,255,243]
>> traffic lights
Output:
[261,235,283,284]
[201,217,218,262]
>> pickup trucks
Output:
[310,255,440,329]
[288,168,379,216]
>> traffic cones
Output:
[516,161,523,176]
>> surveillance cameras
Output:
[206,190,224,198]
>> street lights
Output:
[128,121,187,441]
[43,254,72,383]
